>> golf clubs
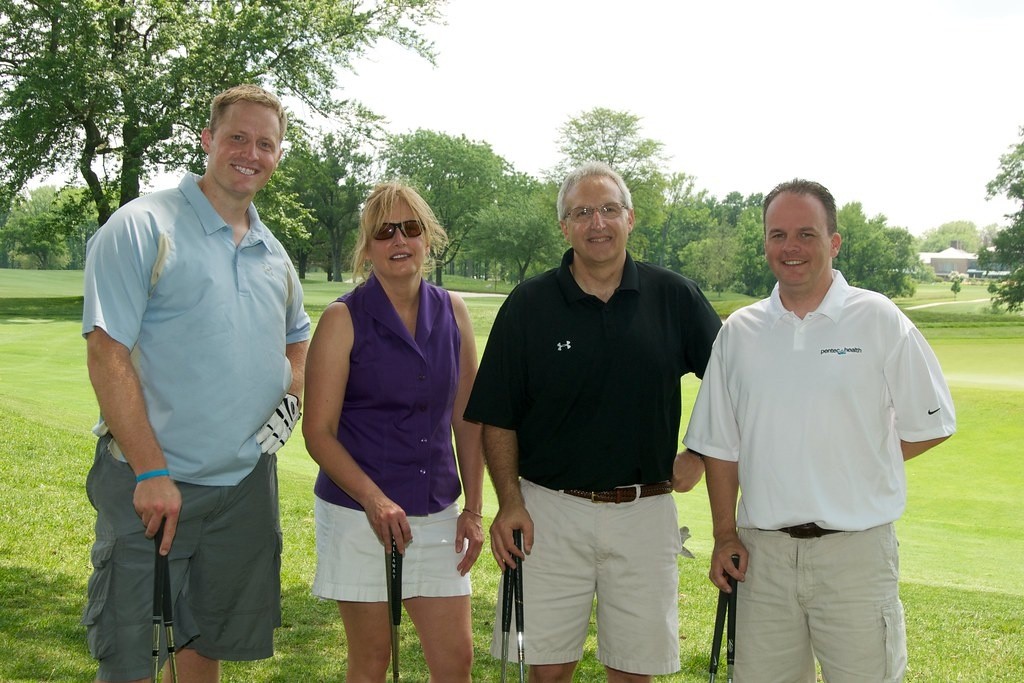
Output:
[153,516,739,683]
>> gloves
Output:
[257,393,301,455]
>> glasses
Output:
[564,202,626,223]
[372,220,424,240]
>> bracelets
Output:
[132,467,172,482]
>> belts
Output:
[778,524,842,539]
[559,482,672,504]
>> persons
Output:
[461,161,726,683]
[80,84,315,683]
[300,183,487,683]
[678,178,958,682]
